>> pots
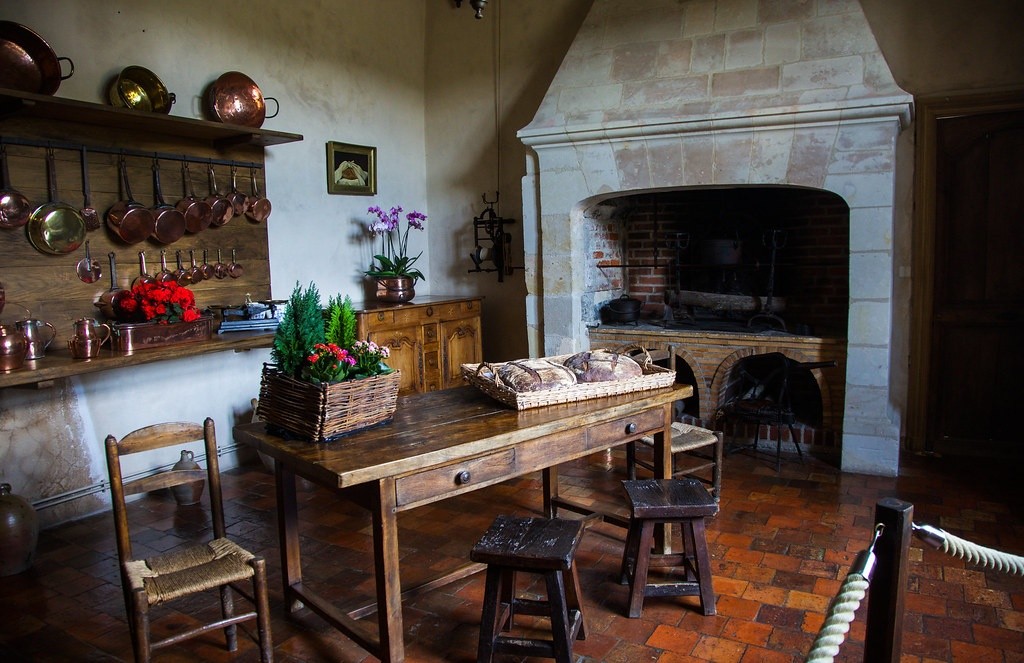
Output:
[208,71,280,128]
[110,65,177,115]
[0,140,272,319]
[0,19,74,97]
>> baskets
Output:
[460,344,676,411]
[256,360,401,441]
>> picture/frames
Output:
[328,141,377,196]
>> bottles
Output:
[0,483,41,565]
[171,450,206,506]
[0,324,28,373]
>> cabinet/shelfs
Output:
[0,86,304,388]
[323,295,487,397]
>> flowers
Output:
[363,204,427,282]
[267,278,394,385]
[112,275,202,324]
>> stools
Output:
[470,514,589,663]
[619,478,720,619]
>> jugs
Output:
[66,318,111,359]
[15,318,56,361]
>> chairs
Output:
[725,351,805,473]
[627,343,722,512]
[105,416,275,663]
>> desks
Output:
[232,384,693,663]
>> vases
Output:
[375,275,418,302]
